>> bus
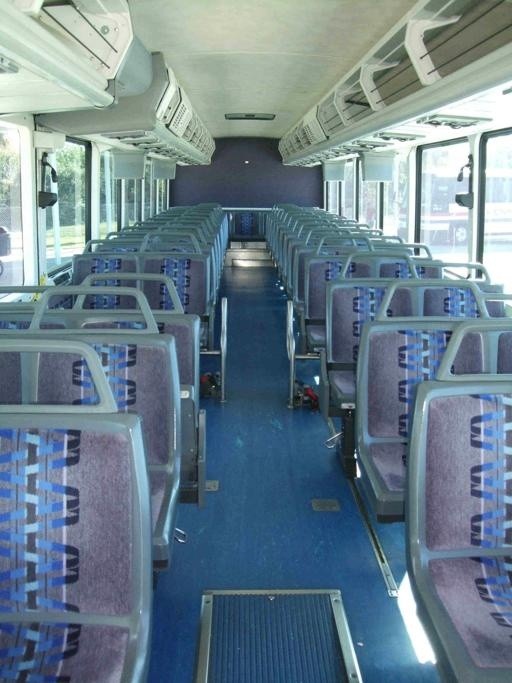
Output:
[398,169,512,245]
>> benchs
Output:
[265,202,512,683]
[0,203,234,681]
